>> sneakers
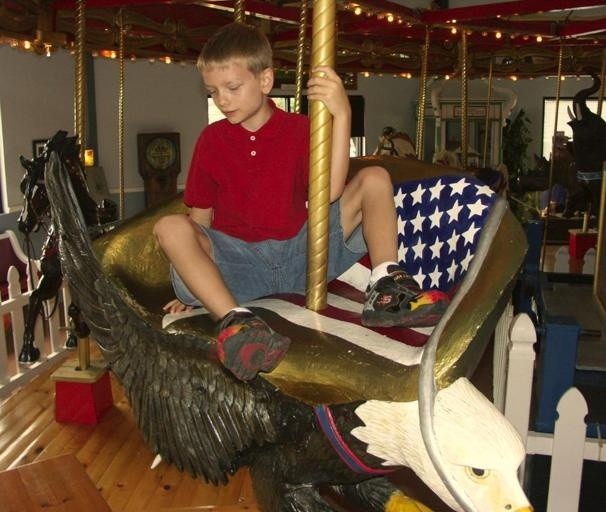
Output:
[358,263,453,329]
[212,309,292,385]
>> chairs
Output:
[0,230,43,334]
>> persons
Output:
[148,19,449,381]
[542,143,568,217]
